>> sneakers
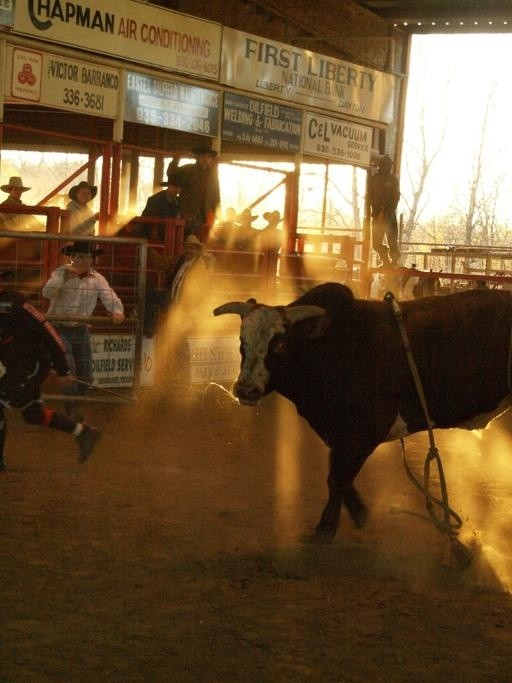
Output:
[76,423,100,465]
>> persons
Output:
[1,176,42,232]
[143,146,283,309]
[1,284,102,471]
[368,155,401,265]
[41,239,127,394]
[69,182,104,237]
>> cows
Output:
[212,287,512,543]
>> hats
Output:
[179,235,204,248]
[235,208,258,222]
[193,145,218,158]
[62,241,104,256]
[160,174,186,187]
[69,181,97,199]
[1,176,31,193]
[264,211,285,223]
